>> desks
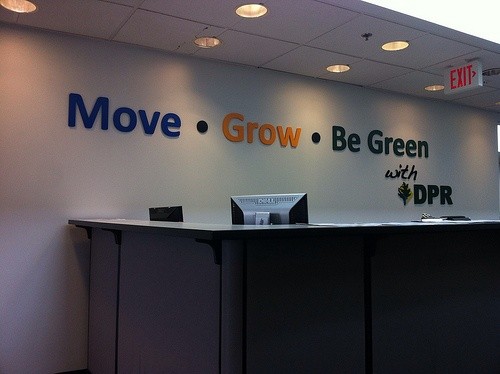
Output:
[72,216,500,374]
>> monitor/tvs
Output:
[148,206,184,222]
[231,193,309,226]
